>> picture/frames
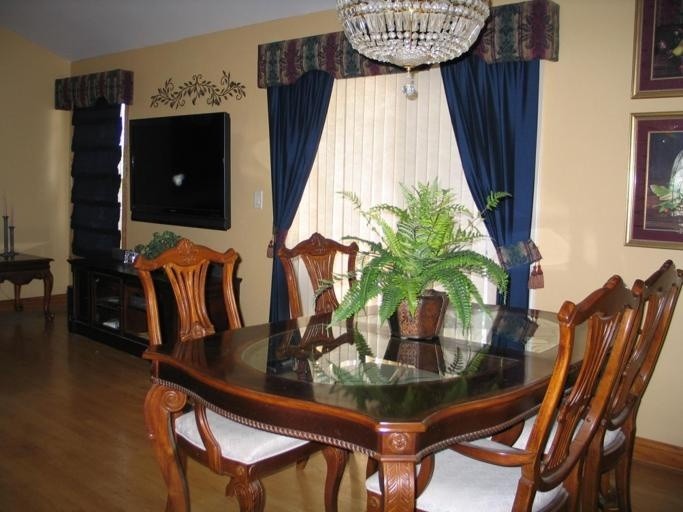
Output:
[620,1,683,251]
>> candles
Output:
[2,194,15,226]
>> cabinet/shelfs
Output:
[66,257,242,361]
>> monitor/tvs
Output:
[129,112,231,231]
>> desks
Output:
[0,252,54,318]
[143,299,644,512]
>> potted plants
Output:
[313,176,513,344]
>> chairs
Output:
[276,232,377,478]
[487,259,682,512]
[365,275,645,512]
[133,237,350,512]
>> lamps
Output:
[335,0,492,99]
